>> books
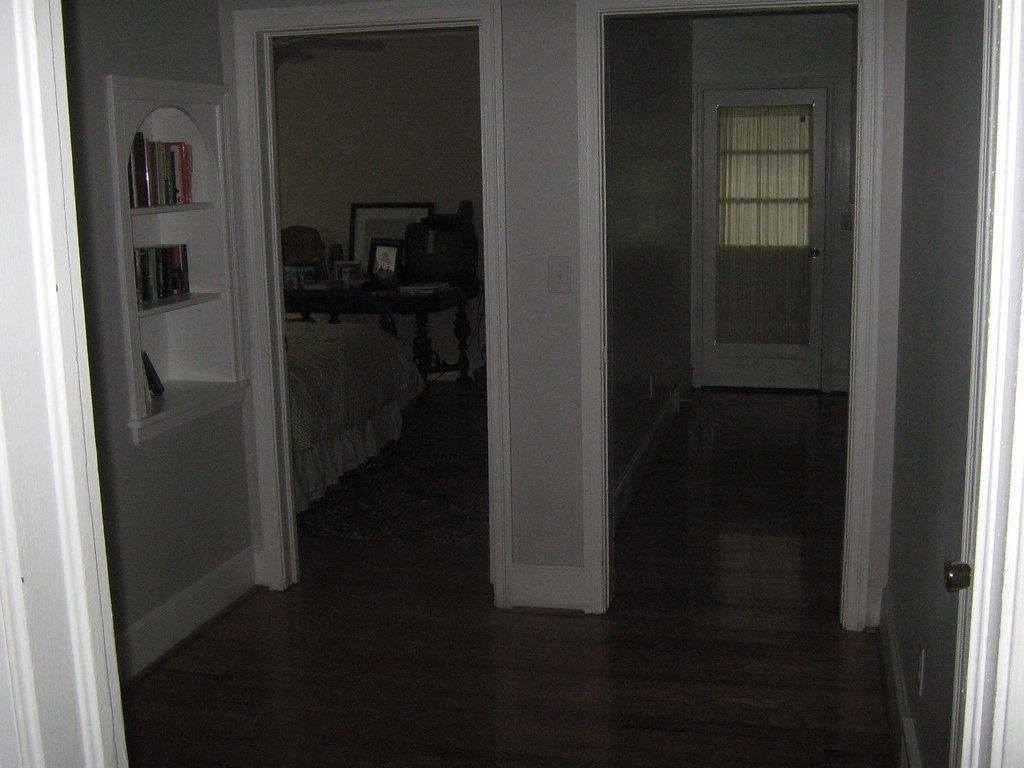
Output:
[400,282,449,297]
[141,350,165,402]
[134,244,190,309]
[127,131,193,208]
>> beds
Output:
[284,322,424,515]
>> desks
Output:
[284,283,480,368]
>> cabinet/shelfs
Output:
[106,76,243,444]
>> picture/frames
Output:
[345,202,433,273]
[367,235,402,276]
